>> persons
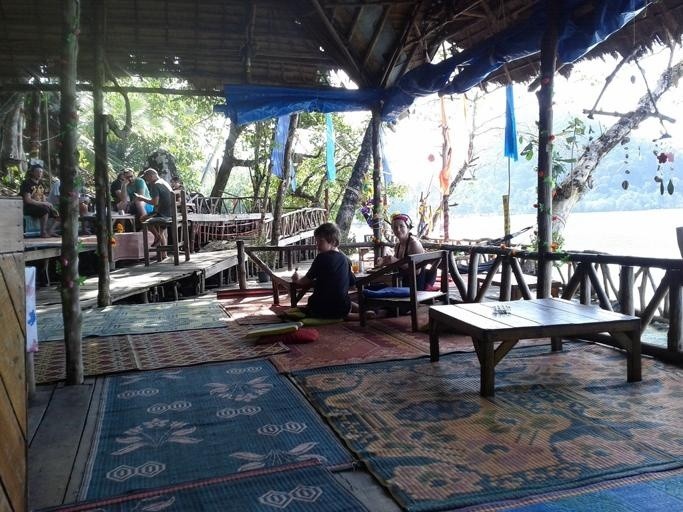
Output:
[289,223,375,320]
[375,213,424,291]
[21,163,183,260]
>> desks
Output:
[80,210,136,235]
[268,259,423,310]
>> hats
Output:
[392,214,412,227]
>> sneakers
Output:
[151,234,162,247]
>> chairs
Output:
[357,246,454,333]
[140,190,190,267]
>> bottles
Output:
[80,201,84,206]
[88,204,93,212]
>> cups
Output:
[351,259,359,273]
[118,209,123,215]
[361,262,370,273]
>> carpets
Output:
[23,293,683,512]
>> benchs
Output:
[22,200,98,237]
